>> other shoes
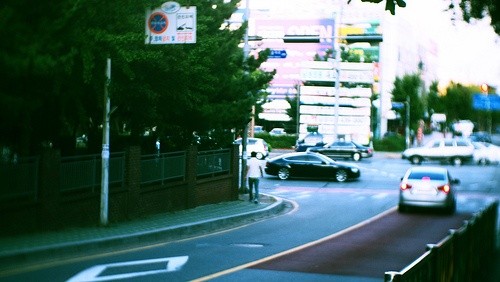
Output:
[254,199,258,204]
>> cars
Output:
[269,128,286,136]
[264,152,360,182]
[398,167,460,215]
[234,138,269,160]
[248,126,264,134]
[306,141,374,162]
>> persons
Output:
[246,152,264,203]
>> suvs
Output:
[402,139,475,166]
